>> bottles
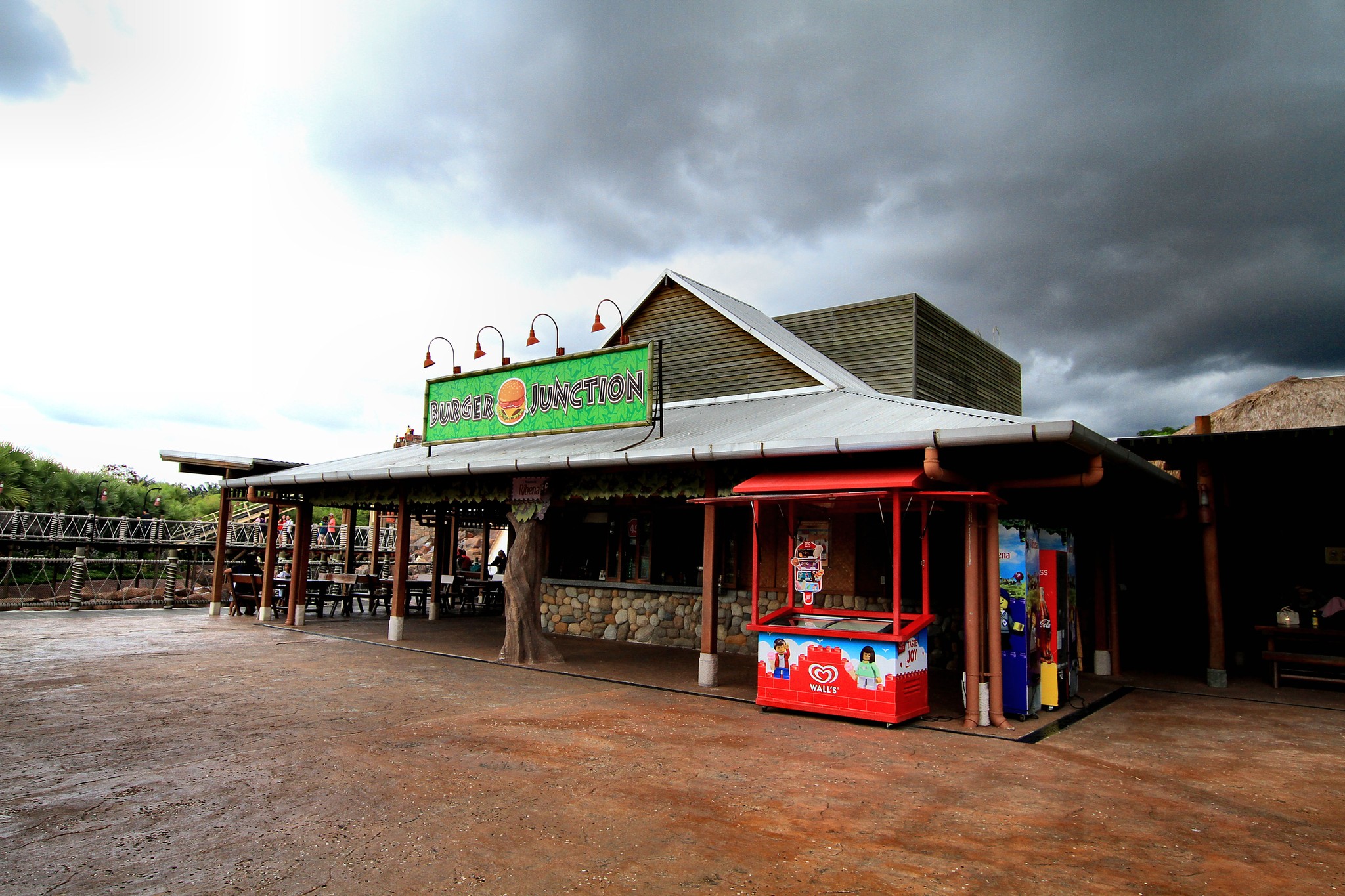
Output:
[1040,587,1051,659]
[1312,610,1318,626]
[679,572,686,585]
[718,575,721,587]
[661,571,665,584]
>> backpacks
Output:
[259,517,266,532]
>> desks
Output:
[1254,623,1345,683]
[363,578,432,617]
[464,577,504,613]
[257,578,334,618]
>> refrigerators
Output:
[747,605,937,729]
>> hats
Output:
[329,513,334,516]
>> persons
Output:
[249,513,266,547]
[327,513,337,549]
[136,509,152,543]
[457,548,490,598]
[228,581,236,615]
[492,550,507,575]
[224,553,264,616]
[276,562,292,613]
[286,514,293,547]
[278,514,287,547]
[317,516,328,549]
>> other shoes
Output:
[228,612,231,615]
[277,609,280,611]
[279,609,284,612]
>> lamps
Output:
[526,314,565,356]
[101,482,107,501]
[473,326,510,366]
[154,489,161,507]
[591,299,630,345]
[423,337,462,375]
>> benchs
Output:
[227,570,507,620]
[1260,650,1345,689]
